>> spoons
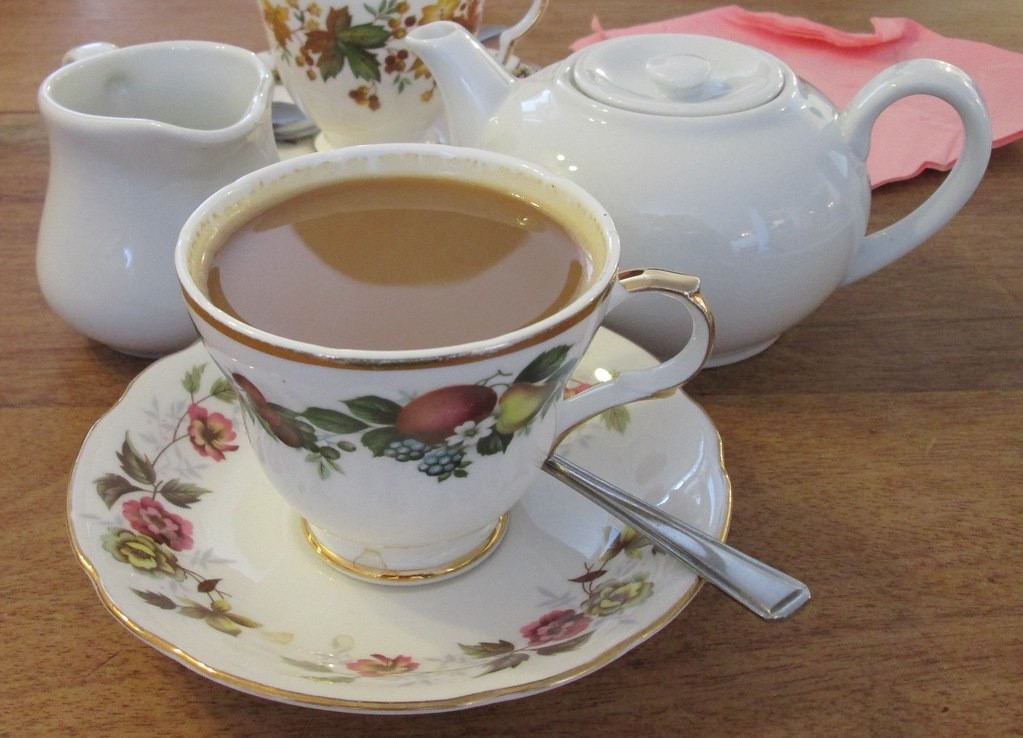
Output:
[271,24,504,143]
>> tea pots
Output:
[404,20,991,370]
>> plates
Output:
[65,327,737,715]
[255,48,539,160]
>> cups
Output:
[173,146,715,589]
[37,41,280,358]
[255,0,548,151]
